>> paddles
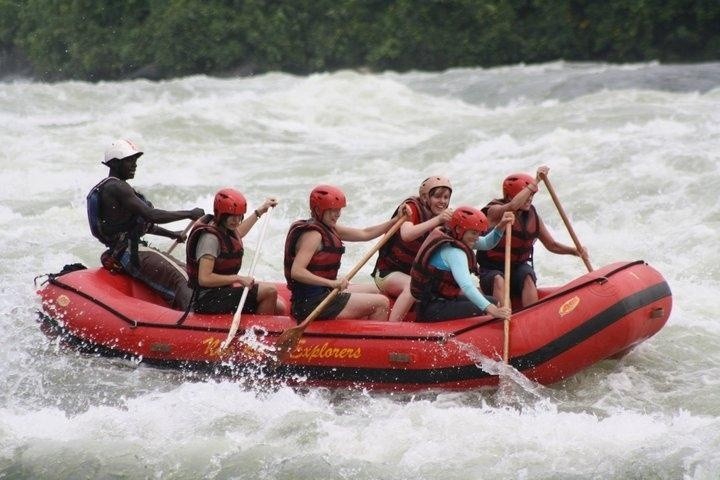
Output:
[265,214,409,371]
[216,208,272,355]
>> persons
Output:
[412,206,514,322]
[100,139,207,314]
[479,164,591,311]
[183,188,287,315]
[373,176,454,323]
[286,185,412,320]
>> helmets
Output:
[310,186,346,222]
[104,139,143,161]
[503,174,538,198]
[214,189,247,221]
[420,177,452,204]
[451,207,487,241]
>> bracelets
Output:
[527,183,536,191]
[254,207,262,221]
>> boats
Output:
[37,260,672,394]
